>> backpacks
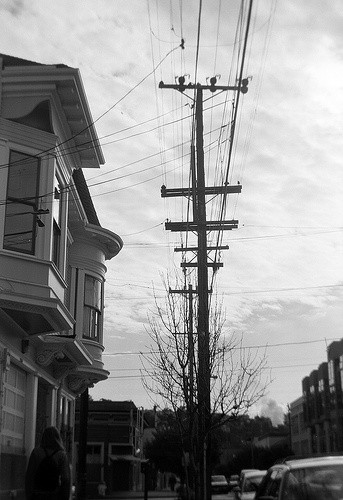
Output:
[38,448,58,486]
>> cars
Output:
[252,455,343,500]
[230,469,262,493]
[211,475,229,494]
[235,470,267,500]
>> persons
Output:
[24,426,71,500]
[168,474,176,491]
